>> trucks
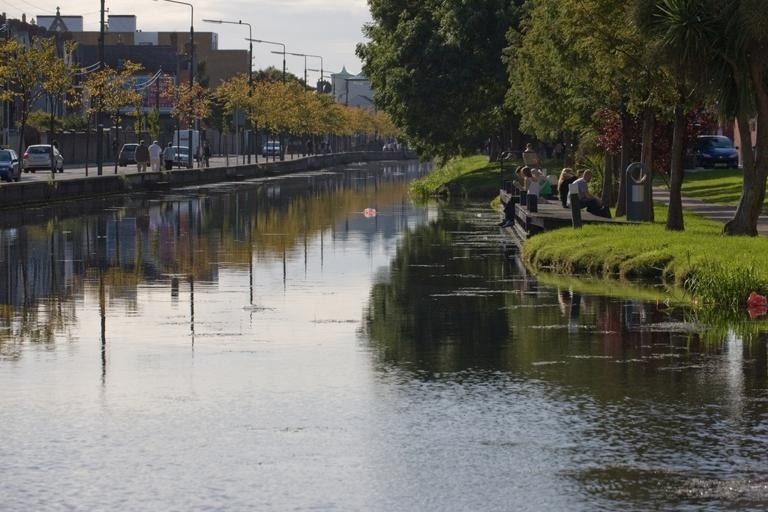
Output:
[172,130,205,161]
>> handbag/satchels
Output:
[600,204,611,218]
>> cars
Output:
[263,141,283,157]
[696,135,739,168]
[1,149,22,182]
[383,143,393,152]
[163,146,193,168]
[118,144,150,167]
[23,144,64,172]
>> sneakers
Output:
[498,219,515,227]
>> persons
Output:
[134,140,149,172]
[498,143,602,228]
[148,140,162,171]
[203,139,212,167]
[163,142,175,170]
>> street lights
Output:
[156,0,193,167]
[245,37,286,160]
[272,51,310,157]
[203,19,252,163]
[293,54,337,99]
